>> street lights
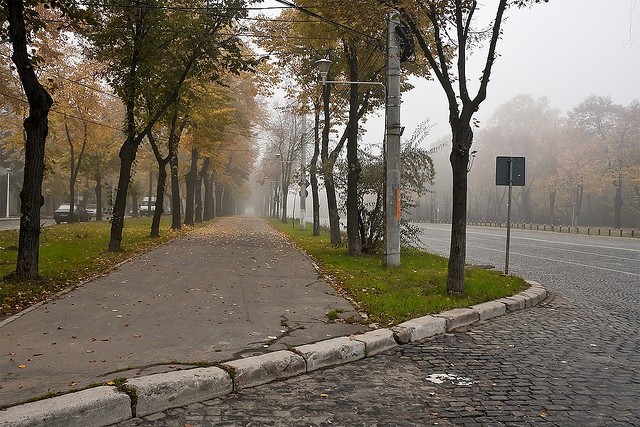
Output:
[315,59,400,268]
[275,154,306,229]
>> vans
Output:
[139,197,157,217]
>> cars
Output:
[85,204,104,217]
[53,204,91,224]
